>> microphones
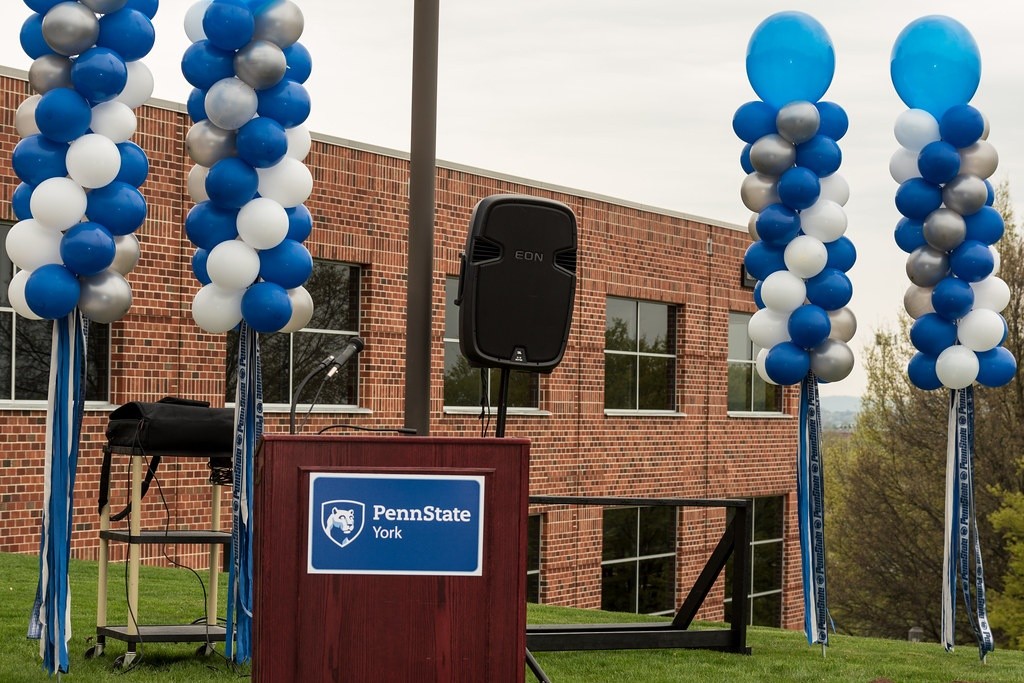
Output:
[317,424,418,435]
[324,337,365,383]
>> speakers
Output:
[454,193,578,374]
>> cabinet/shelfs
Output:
[85,444,237,673]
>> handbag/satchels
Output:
[97,396,235,521]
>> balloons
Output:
[733,102,856,385]
[889,14,981,119]
[888,105,1018,388]
[180,0,315,333]
[4,0,161,325]
[745,11,835,110]
